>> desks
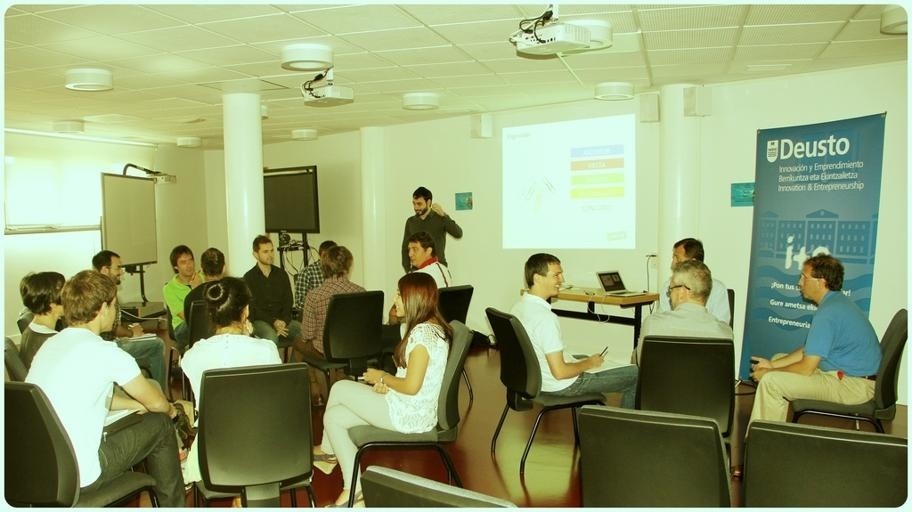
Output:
[520,286,661,346]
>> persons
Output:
[402,187,463,275]
[636,260,733,368]
[652,239,731,326]
[510,253,640,410]
[742,256,883,441]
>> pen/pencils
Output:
[600,346,608,356]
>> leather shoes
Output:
[731,465,744,481]
[312,394,324,407]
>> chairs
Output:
[387,285,475,400]
[342,320,475,508]
[577,404,732,505]
[741,419,906,506]
[166,300,211,400]
[18,308,34,334]
[6,336,160,506]
[727,289,735,332]
[193,362,317,507]
[485,307,607,475]
[301,290,384,401]
[634,336,736,438]
[792,308,908,433]
[361,466,517,507]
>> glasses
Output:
[666,284,691,297]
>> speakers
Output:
[683,86,710,116]
[472,114,491,138]
[639,93,659,122]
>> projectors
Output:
[304,86,354,108]
[515,23,591,55]
[149,176,176,184]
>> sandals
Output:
[324,490,365,508]
[312,454,338,475]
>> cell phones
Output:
[599,346,608,358]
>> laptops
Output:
[596,270,646,297]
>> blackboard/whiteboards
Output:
[101,173,157,267]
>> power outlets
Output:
[644,251,658,261]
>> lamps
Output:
[65,68,113,91]
[261,105,269,119]
[565,21,612,50]
[595,83,631,101]
[280,43,334,71]
[292,129,319,141]
[878,10,907,36]
[177,137,201,147]
[402,93,439,110]
